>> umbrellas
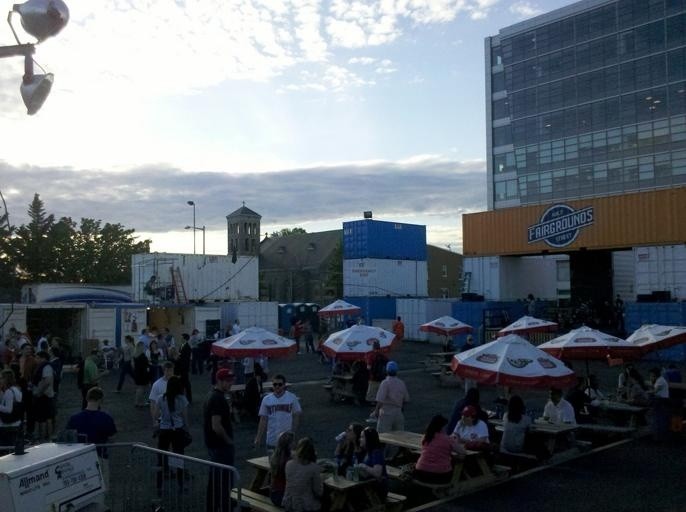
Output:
[606,323,686,368]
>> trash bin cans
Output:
[564,433,605,492]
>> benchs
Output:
[230,349,685,512]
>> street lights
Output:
[186,201,195,254]
[440,287,447,298]
[184,225,205,255]
[0,0,69,116]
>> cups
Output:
[267,447,275,464]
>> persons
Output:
[2,328,268,511]
[289,317,328,351]
[443,336,512,400]
[233,319,242,333]
[251,341,410,511]
[393,316,404,340]
[358,315,366,324]
[518,294,626,337]
[411,360,669,512]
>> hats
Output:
[386,361,399,372]
[460,405,477,417]
[492,395,508,406]
[215,368,237,381]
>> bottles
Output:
[524,407,536,424]
[344,458,359,484]
[606,394,617,407]
[332,462,340,485]
[494,404,505,419]
[334,430,346,443]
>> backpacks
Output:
[370,353,388,382]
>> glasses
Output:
[272,383,284,387]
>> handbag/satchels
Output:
[0,387,24,424]
[172,424,193,450]
[464,440,493,451]
[398,462,417,483]
